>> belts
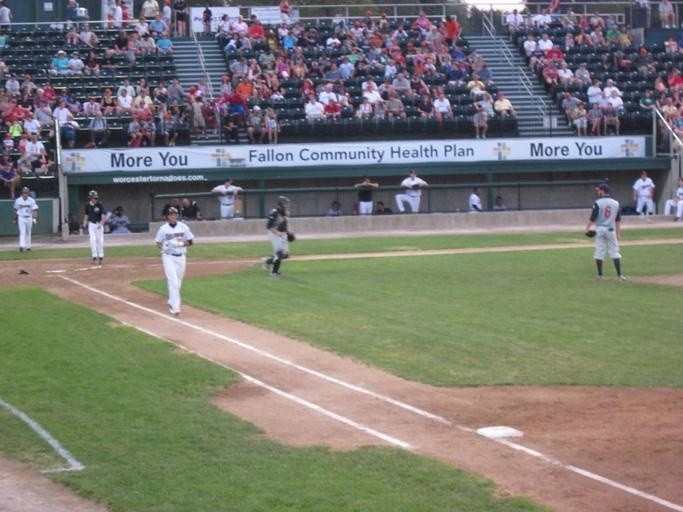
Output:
[173,254,181,256]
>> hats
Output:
[595,184,608,192]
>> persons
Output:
[216,1,518,148]
[108,206,135,234]
[470,186,509,211]
[501,0,683,156]
[262,196,289,276]
[210,175,243,220]
[12,186,38,251]
[161,199,202,220]
[584,184,626,281]
[82,191,107,263]
[1,1,216,199]
[632,170,683,222]
[155,207,193,316]
[326,169,429,215]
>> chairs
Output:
[512,27,682,139]
[218,19,518,141]
[0,12,192,183]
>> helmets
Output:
[167,207,178,213]
[88,190,98,199]
[278,196,290,205]
